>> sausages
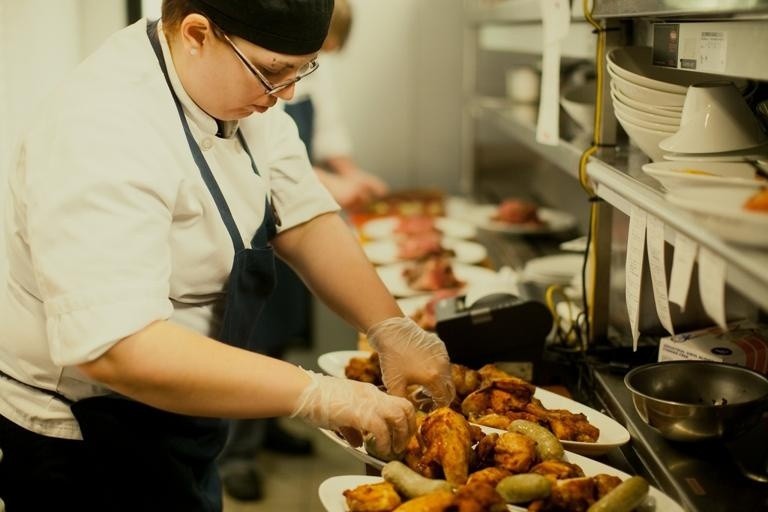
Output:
[587,475,649,512]
[364,432,404,461]
[495,474,551,503]
[381,460,452,498]
[508,419,564,461]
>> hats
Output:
[188,0,335,56]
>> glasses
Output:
[222,31,323,99]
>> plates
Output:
[640,160,767,250]
[307,341,686,510]
[358,200,585,311]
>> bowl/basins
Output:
[657,78,764,156]
[604,45,688,161]
[622,358,766,446]
[559,78,595,134]
[504,69,541,107]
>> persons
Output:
[207,0,386,502]
[0,1,457,510]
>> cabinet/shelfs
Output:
[458,0,766,512]
